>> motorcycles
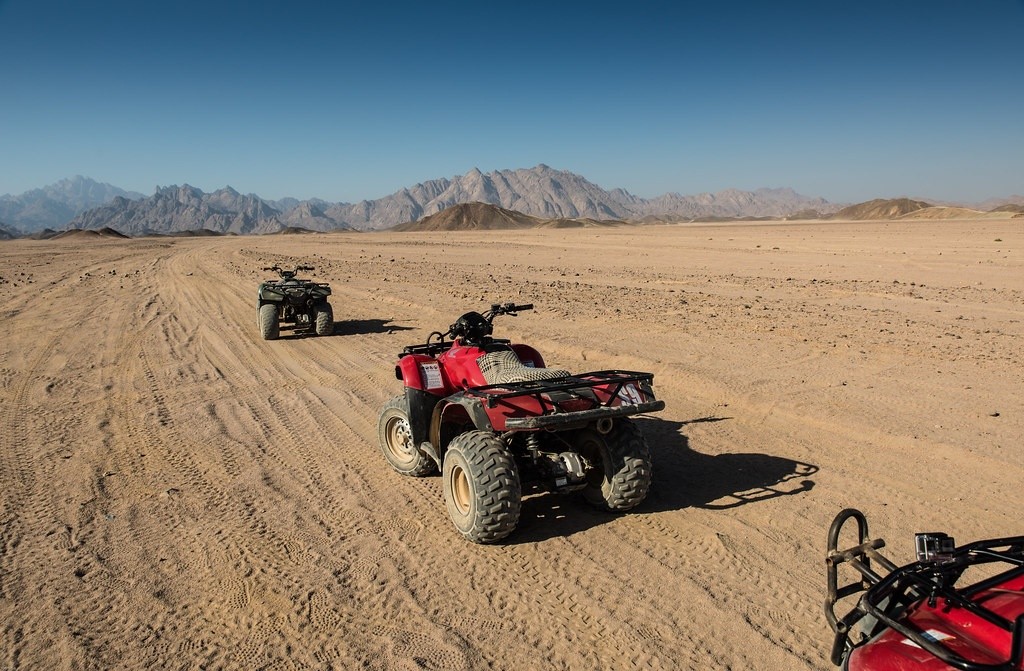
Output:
[822,507,1024,671]
[255,265,335,340]
[377,300,666,546]
